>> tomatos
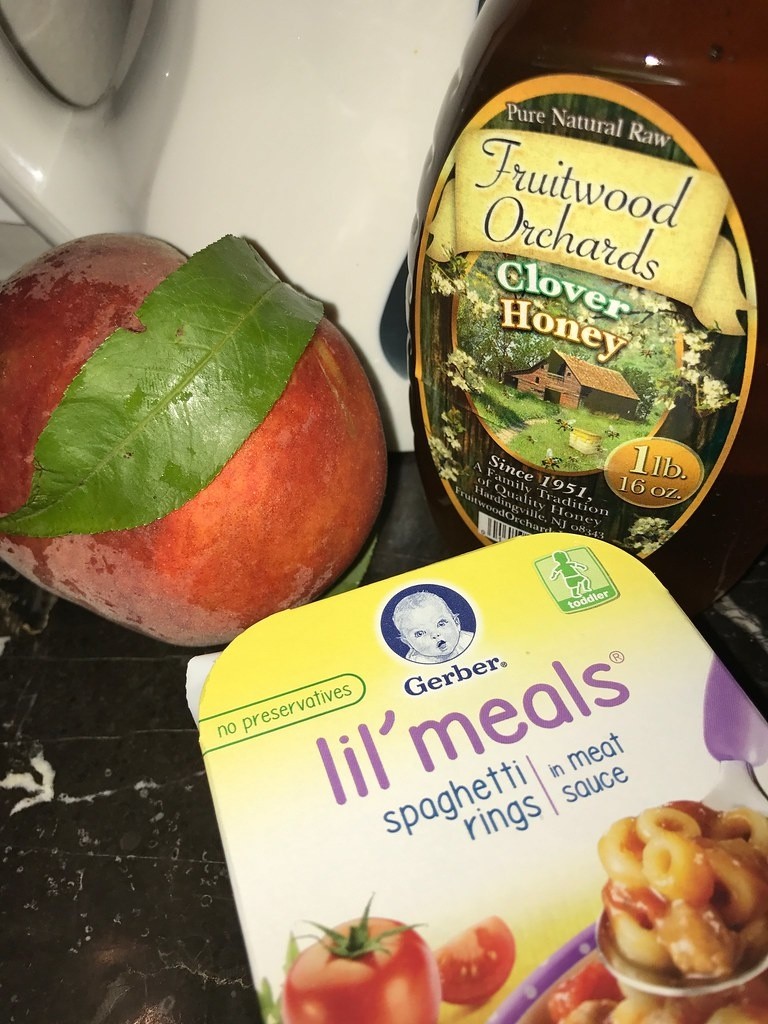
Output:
[283,892,520,1024]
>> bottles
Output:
[402,0,768,619]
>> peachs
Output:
[0,231,392,650]
